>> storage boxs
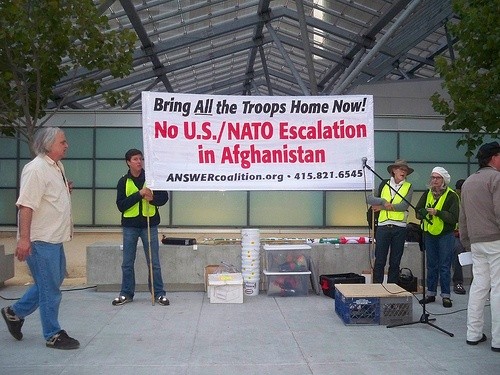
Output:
[207,273,244,304]
[319,273,365,299]
[262,243,312,297]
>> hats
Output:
[431,167,450,185]
[387,160,414,176]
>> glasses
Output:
[431,176,442,180]
[395,168,409,173]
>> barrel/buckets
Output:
[241,229,260,297]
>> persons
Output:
[416,167,460,308]
[459,141,500,352]
[112,149,169,305]
[366,159,414,286]
[452,179,465,295]
[1,127,80,350]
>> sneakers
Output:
[442,298,452,307]
[419,296,435,304]
[491,347,500,352]
[46,330,79,350]
[454,284,466,294]
[112,295,133,305]
[156,296,169,306]
[467,333,487,345]
[1,306,25,340]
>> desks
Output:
[87,237,472,293]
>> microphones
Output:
[361,157,367,168]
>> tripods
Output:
[367,166,455,336]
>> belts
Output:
[384,224,396,228]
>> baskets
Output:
[335,287,380,327]
[380,297,413,325]
